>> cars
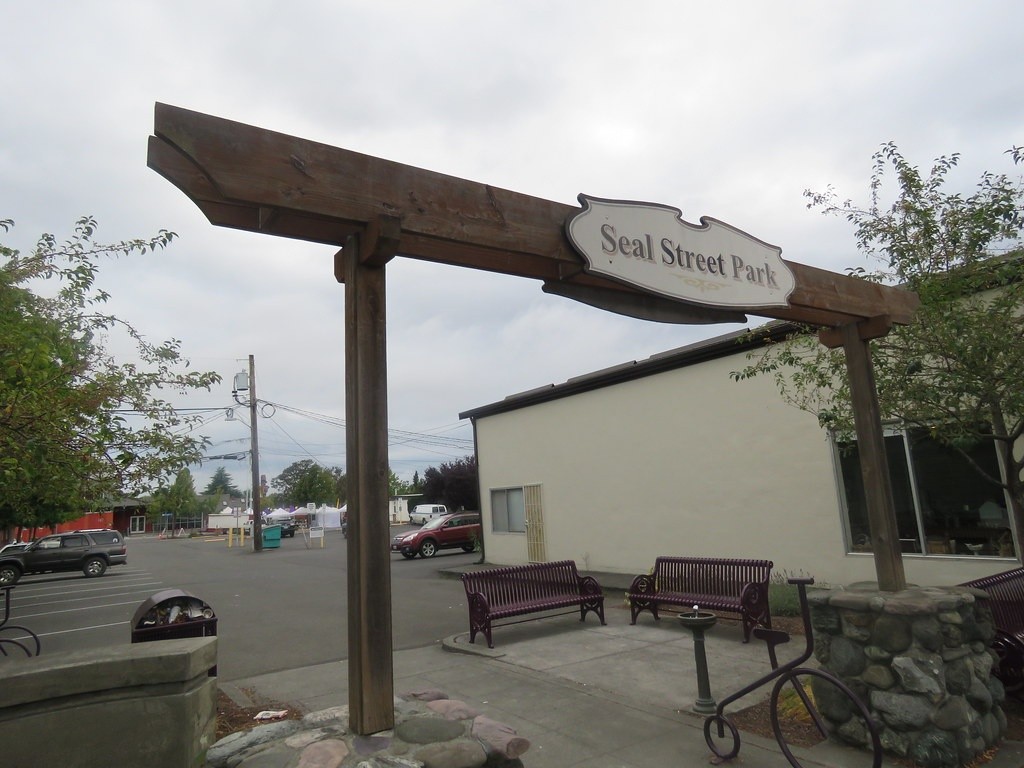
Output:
[0,540,62,557]
[274,520,299,538]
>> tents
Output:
[219,503,349,530]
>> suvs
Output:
[390,510,482,560]
[0,529,128,588]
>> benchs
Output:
[626,556,775,643]
[946,566,1024,714]
[461,560,614,650]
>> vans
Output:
[409,503,447,527]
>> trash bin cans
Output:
[261,524,282,548]
[130,588,218,676]
